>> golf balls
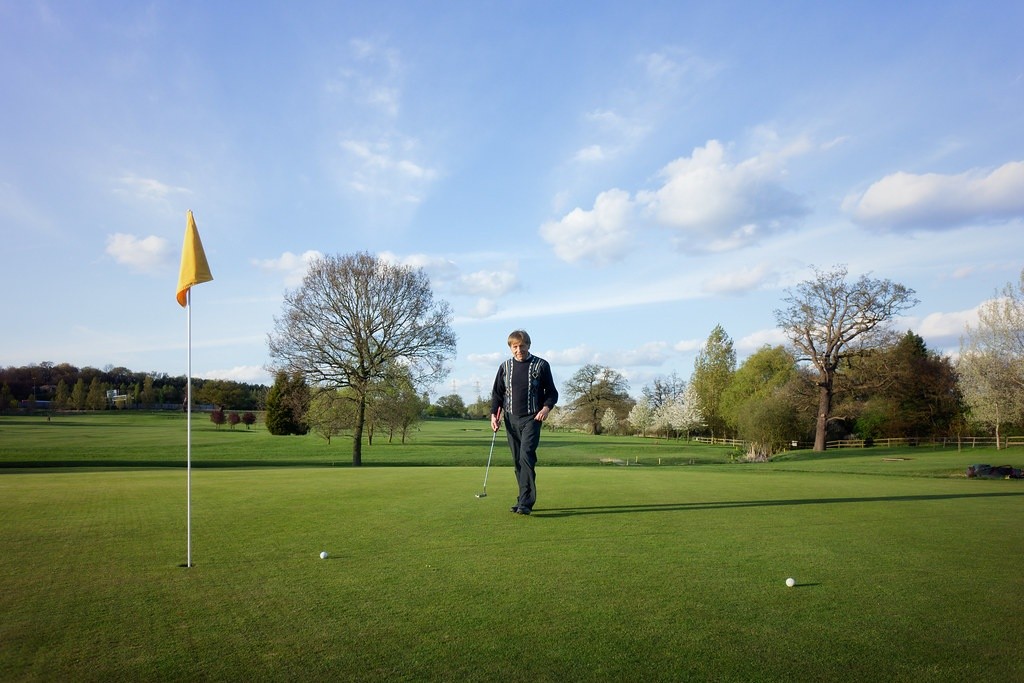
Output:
[786,577,795,587]
[320,551,327,559]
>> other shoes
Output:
[510,505,518,512]
[516,506,530,515]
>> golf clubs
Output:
[473,406,504,498]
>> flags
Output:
[176,213,214,308]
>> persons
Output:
[183,398,188,412]
[491,331,558,515]
